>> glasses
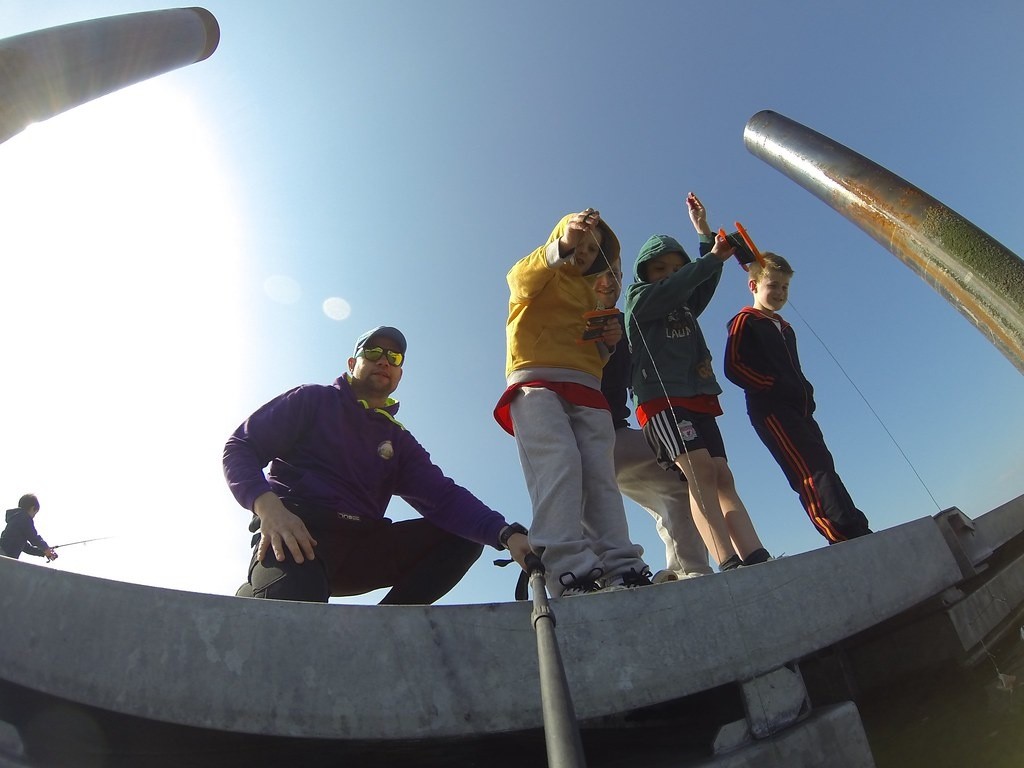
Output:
[355,345,404,366]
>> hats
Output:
[353,326,407,359]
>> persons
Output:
[723,251,875,546]
[627,192,787,574]
[221,326,536,605]
[0,493,58,561]
[492,208,678,595]
[590,256,716,586]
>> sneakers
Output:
[559,565,678,597]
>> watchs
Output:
[500,522,530,546]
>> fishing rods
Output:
[49,537,109,548]
